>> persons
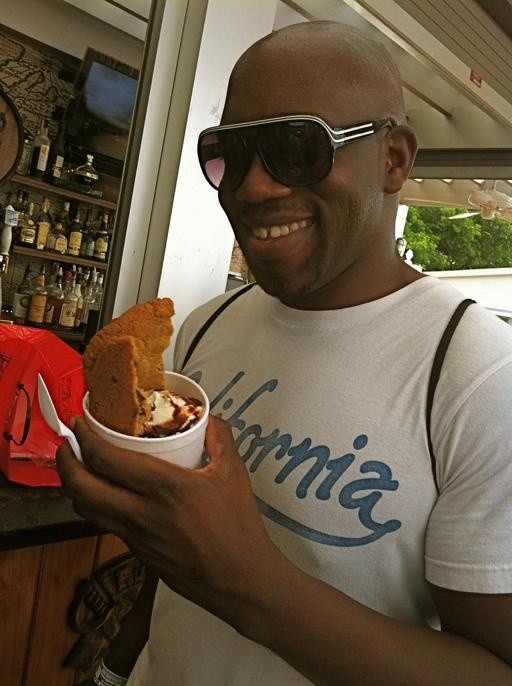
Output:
[56,19,512,685]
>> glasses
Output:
[195,112,400,195]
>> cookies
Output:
[81,298,175,393]
[88,336,152,437]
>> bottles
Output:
[11,118,115,333]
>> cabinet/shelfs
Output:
[1,174,117,342]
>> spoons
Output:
[37,372,84,464]
